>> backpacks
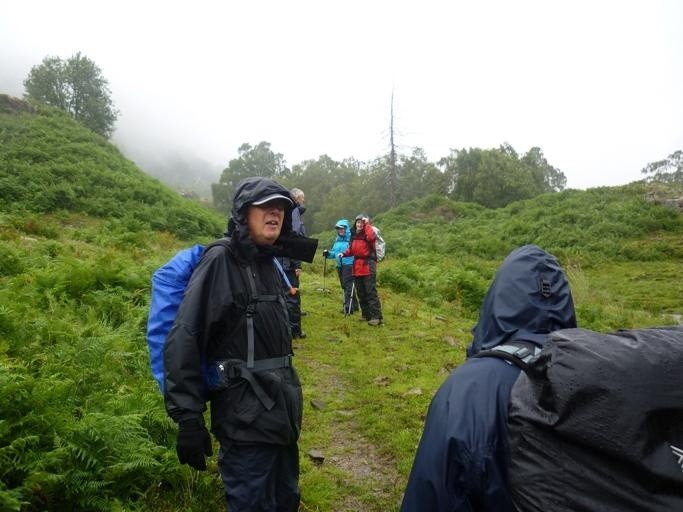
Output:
[147,238,258,402]
[476,328,683,511]
[372,225,385,262]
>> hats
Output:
[250,193,295,207]
[335,225,344,229]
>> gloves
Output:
[323,251,328,256]
[177,416,212,471]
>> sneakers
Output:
[301,333,306,338]
[359,318,383,325]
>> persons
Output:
[162,176,304,512]
[323,219,359,314]
[397,243,578,512]
[276,187,308,339]
[337,213,383,327]
